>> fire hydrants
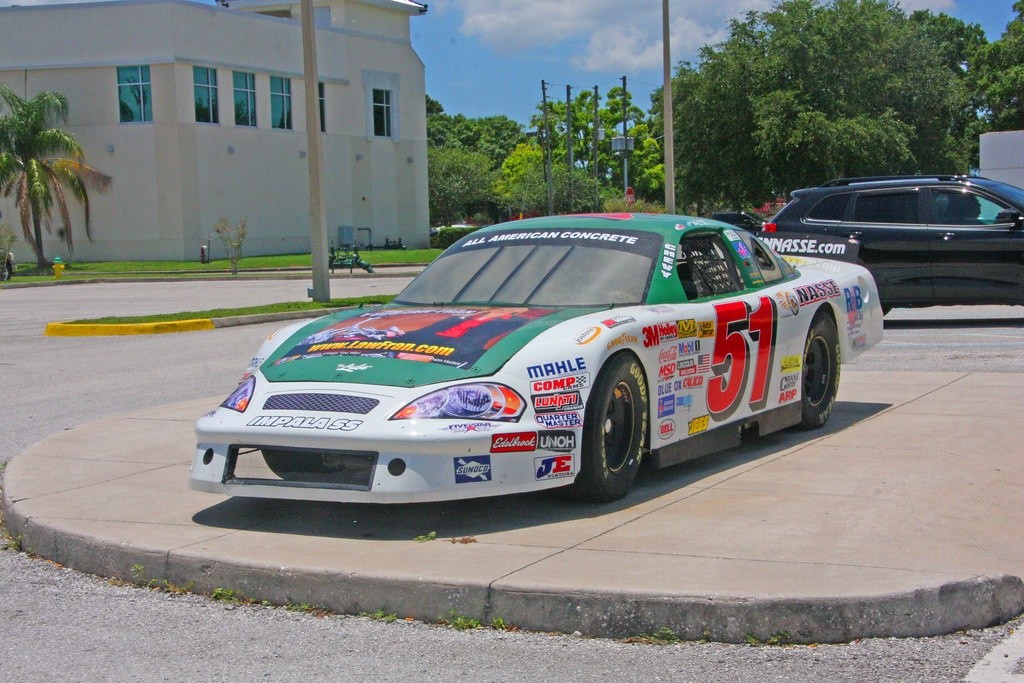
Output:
[52,257,64,280]
[199,246,206,263]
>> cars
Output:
[0,249,17,281]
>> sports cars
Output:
[188,212,883,506]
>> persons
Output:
[964,195,987,227]
[5,252,14,280]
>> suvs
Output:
[702,212,762,236]
[760,174,1024,315]
